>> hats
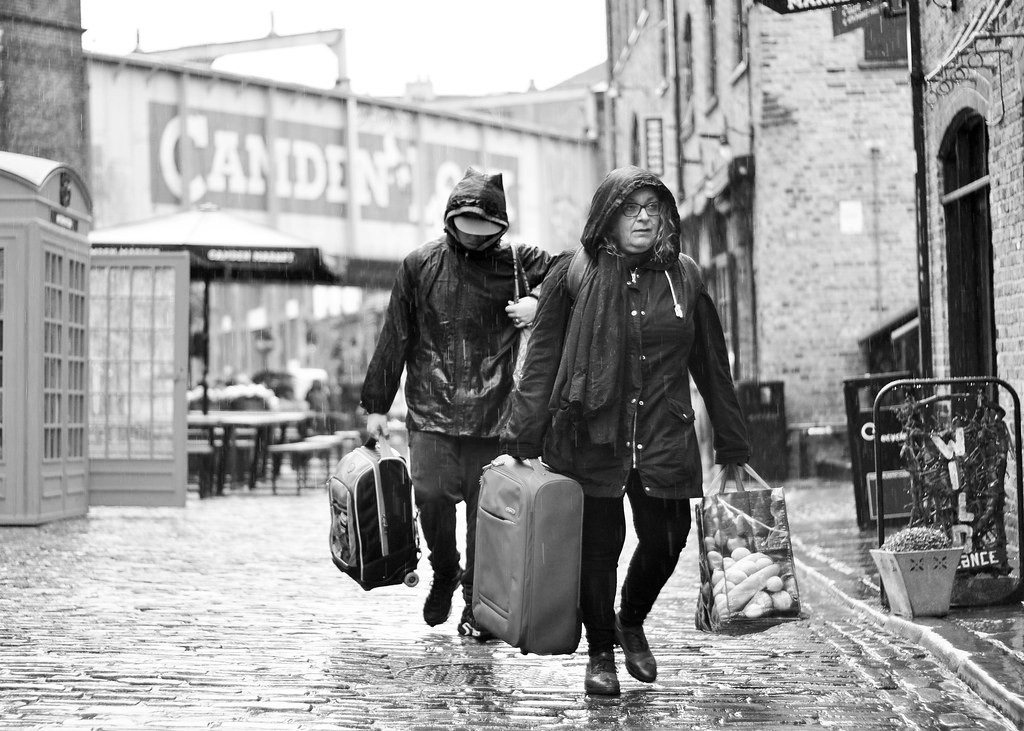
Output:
[454,212,503,235]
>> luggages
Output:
[327,435,421,592]
[472,458,584,656]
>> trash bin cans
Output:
[842,371,925,533]
[723,381,788,482]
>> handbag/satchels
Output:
[695,462,803,636]
[510,244,532,392]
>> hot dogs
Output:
[709,554,780,617]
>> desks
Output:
[187,410,307,498]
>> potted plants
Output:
[870,527,964,618]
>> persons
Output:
[504,163,755,698]
[306,379,330,413]
[359,165,552,644]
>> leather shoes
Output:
[613,613,657,683]
[585,651,620,694]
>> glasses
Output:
[621,201,664,217]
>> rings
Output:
[515,319,519,323]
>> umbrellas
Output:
[87,202,345,416]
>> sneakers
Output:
[424,565,464,626]
[458,604,475,637]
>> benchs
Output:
[187,441,256,499]
[270,431,359,497]
